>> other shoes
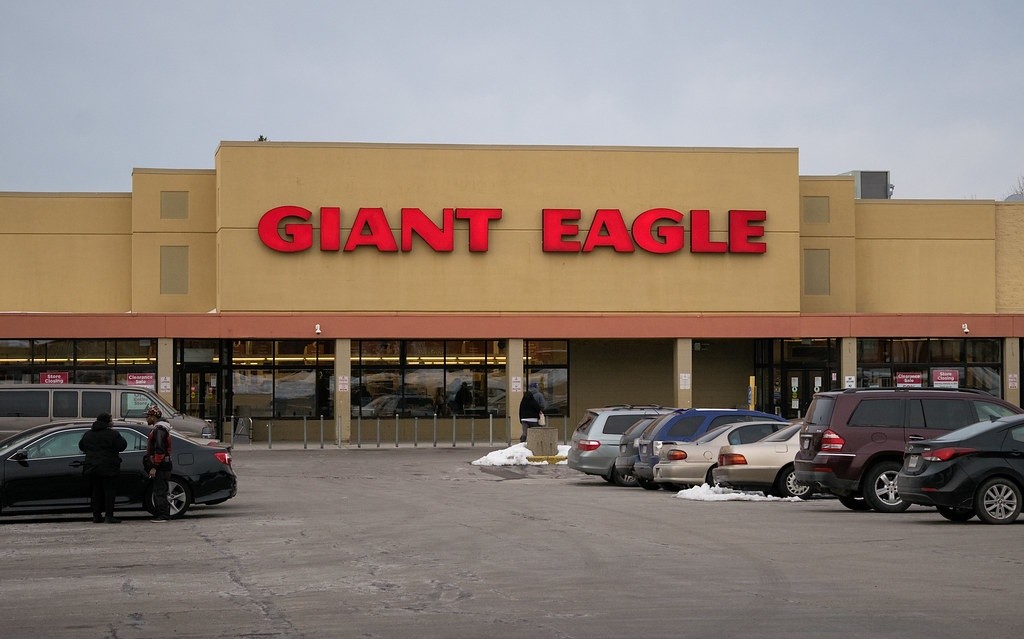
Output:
[104,517,122,523]
[93,517,105,523]
[150,517,168,523]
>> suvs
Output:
[614,413,668,491]
[351,393,438,419]
[633,408,787,492]
[565,404,687,488]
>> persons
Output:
[143,406,172,523]
[518,383,546,442]
[78,412,127,524]
[433,387,446,416]
[454,382,473,414]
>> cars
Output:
[463,385,567,418]
[0,420,233,504]
[0,424,238,520]
[712,424,815,499]
[896,412,1024,525]
[653,421,792,489]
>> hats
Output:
[144,404,162,417]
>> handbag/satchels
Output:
[537,410,546,426]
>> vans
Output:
[794,387,1023,513]
[0,383,219,452]
